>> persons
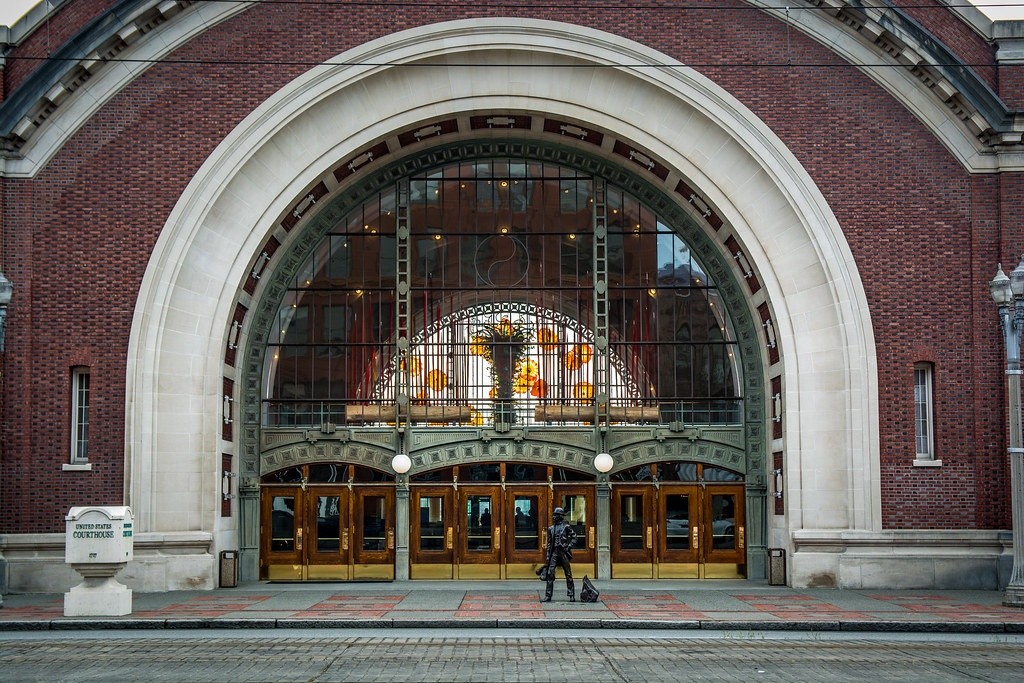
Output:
[540,507,578,602]
[515,507,524,528]
[480,508,491,526]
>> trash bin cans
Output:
[220,549,239,588]
[767,547,786,586]
